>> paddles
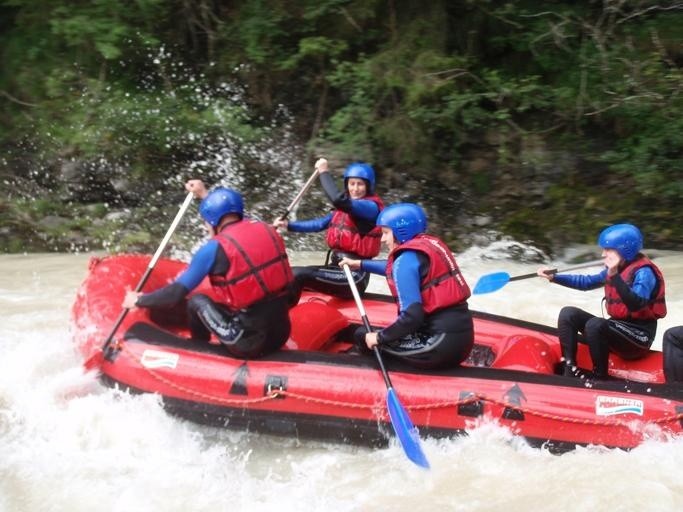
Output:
[343,263,431,469]
[82,192,194,377]
[473,259,606,295]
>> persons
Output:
[273,155,383,303]
[335,202,474,370]
[121,179,295,359]
[532,220,666,380]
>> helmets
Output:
[376,202,427,245]
[344,162,376,197]
[598,222,644,263]
[199,188,245,228]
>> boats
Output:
[72,253,683,455]
